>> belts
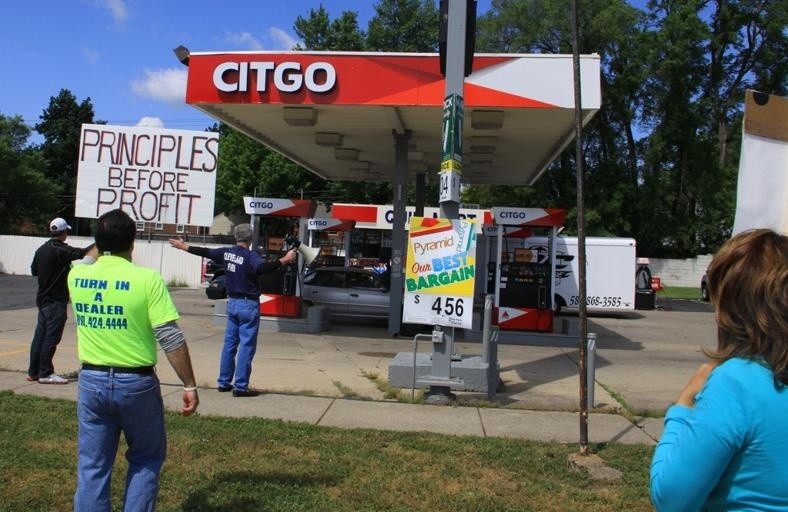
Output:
[81,363,154,374]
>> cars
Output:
[301,265,404,319]
[202,247,296,300]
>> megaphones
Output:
[284,234,322,266]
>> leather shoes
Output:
[218,385,257,397]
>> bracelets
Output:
[183,385,198,392]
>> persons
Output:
[27,217,95,384]
[65,208,201,511]
[168,223,298,397]
[647,227,788,511]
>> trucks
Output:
[475,233,636,318]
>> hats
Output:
[234,223,253,242]
[50,217,72,232]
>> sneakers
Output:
[26,372,68,384]
